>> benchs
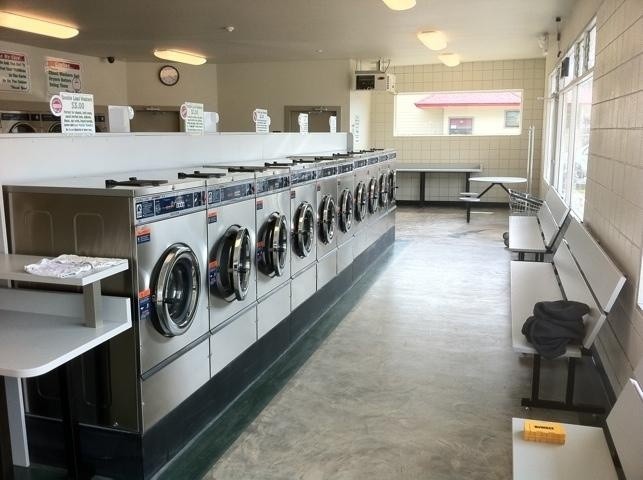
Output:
[505,216,631,414]
[506,183,571,261]
[508,378,643,480]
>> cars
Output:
[563,143,588,178]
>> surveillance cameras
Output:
[108,57,114,63]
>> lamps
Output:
[150,48,208,68]
[415,29,447,51]
[437,53,466,68]
[380,0,420,14]
[0,10,80,43]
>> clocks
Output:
[156,65,179,88]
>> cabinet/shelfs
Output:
[0,248,134,471]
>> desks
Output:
[394,162,481,210]
[465,175,527,224]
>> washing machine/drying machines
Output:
[0,110,110,133]
[2,148,398,434]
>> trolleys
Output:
[502,188,547,249]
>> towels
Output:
[22,251,127,278]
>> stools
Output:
[458,196,480,224]
[457,192,477,198]
